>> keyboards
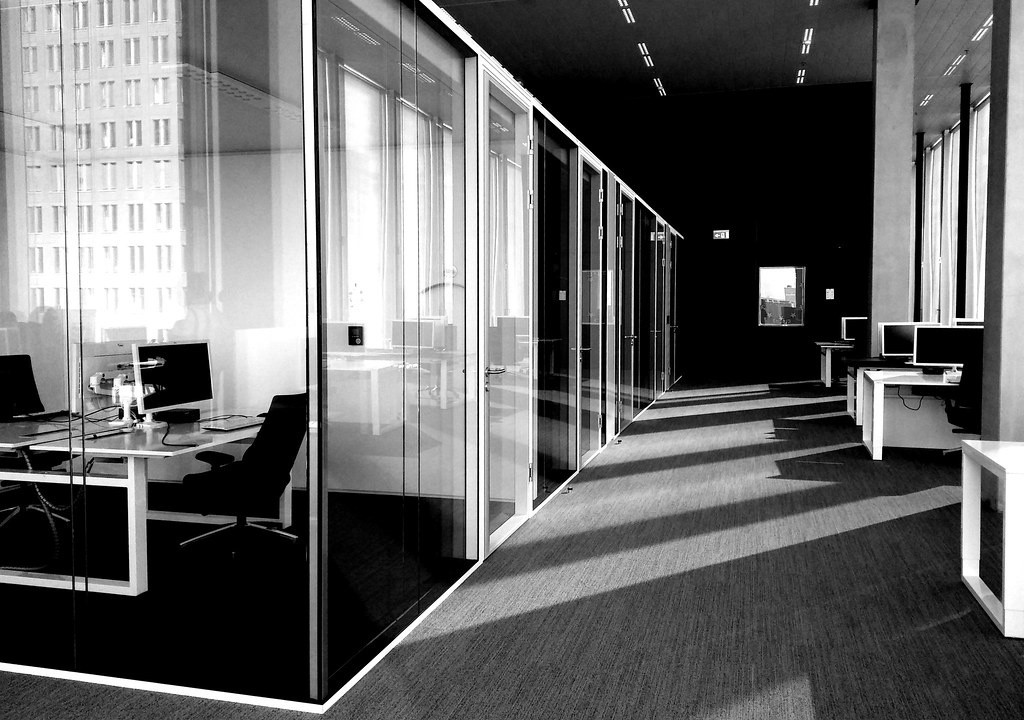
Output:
[203,418,265,432]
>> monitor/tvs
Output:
[132,343,214,428]
[841,316,986,372]
[70,339,148,428]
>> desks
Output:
[847,358,981,461]
[959,438,1024,640]
[815,342,853,387]
[0,410,293,596]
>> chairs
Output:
[943,358,983,457]
[179,391,307,547]
[0,354,81,527]
